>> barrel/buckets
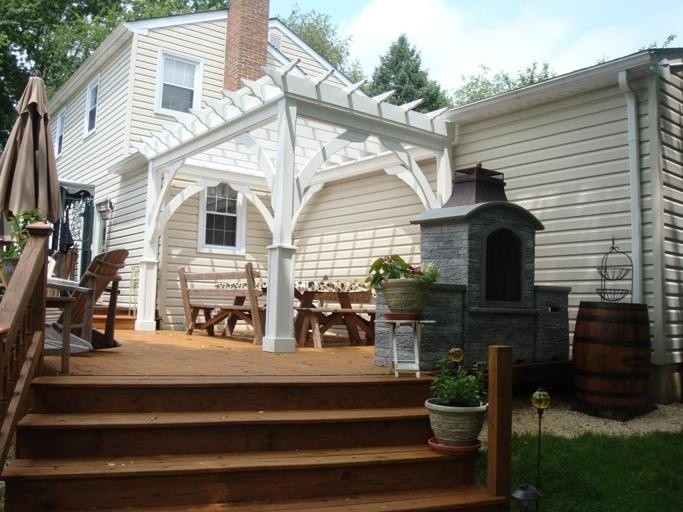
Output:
[572,301,652,420]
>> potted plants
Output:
[365,254,440,320]
[0,209,47,290]
[423,353,491,456]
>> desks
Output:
[214,279,374,348]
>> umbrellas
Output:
[0,74,64,232]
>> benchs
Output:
[292,308,375,350]
[174,263,266,346]
